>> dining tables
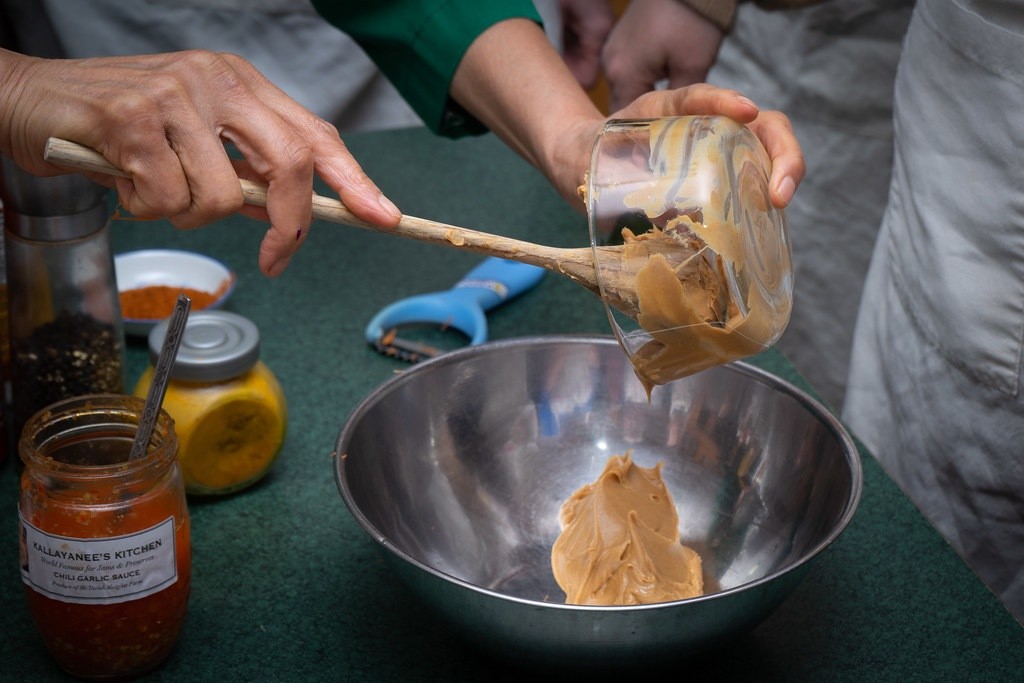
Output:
[1,109,1023,681]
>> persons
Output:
[1,1,808,278]
[600,3,1021,605]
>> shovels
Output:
[43,132,738,334]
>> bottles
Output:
[4,194,126,463]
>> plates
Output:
[114,249,237,336]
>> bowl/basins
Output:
[333,332,862,681]
[588,111,791,386]
